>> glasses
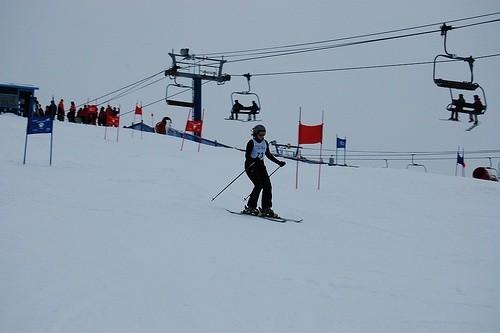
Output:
[258,131,265,136]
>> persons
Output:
[241,124,286,216]
[40,108,43,117]
[82,105,89,124]
[106,105,111,112]
[67,112,71,122]
[45,106,50,115]
[231,99,244,120]
[70,102,76,123]
[449,94,466,121]
[49,100,57,120]
[467,95,483,124]
[98,107,105,126]
[58,99,65,121]
[77,108,83,119]
[110,106,119,127]
[247,100,258,120]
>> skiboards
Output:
[243,120,262,122]
[465,123,478,131]
[226,205,303,222]
[224,118,242,121]
[439,118,461,122]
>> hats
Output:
[254,125,265,133]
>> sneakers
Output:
[245,207,262,216]
[262,208,278,218]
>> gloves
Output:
[254,157,259,163]
[280,161,284,166]
